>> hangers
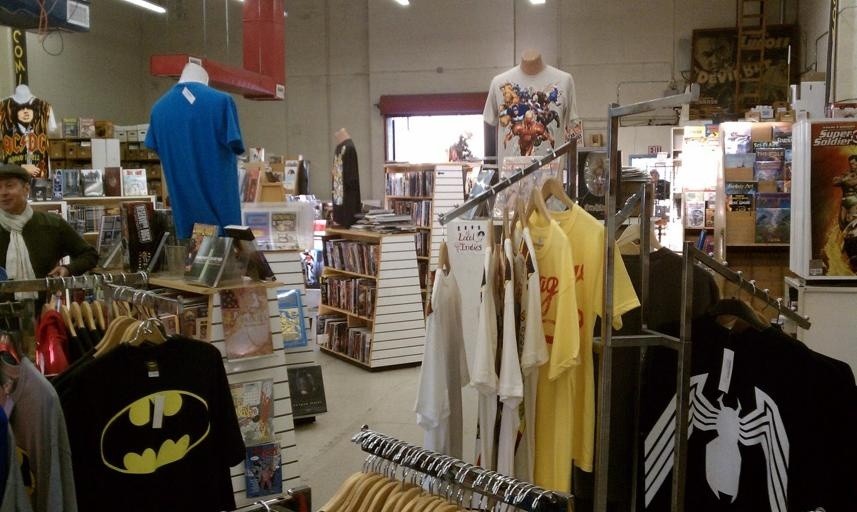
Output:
[317,430,556,511]
[437,147,575,277]
[1,270,168,366]
[705,258,783,339]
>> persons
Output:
[0,164,98,329]
[649,169,670,200]
[831,155,857,232]
[144,64,244,239]
[694,33,723,72]
[333,129,363,228]
[483,50,582,183]
[3,104,43,178]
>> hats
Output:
[1,163,31,182]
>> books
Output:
[245,441,282,498]
[682,122,792,244]
[184,224,277,289]
[287,365,328,417]
[319,171,433,364]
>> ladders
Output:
[735,0,766,116]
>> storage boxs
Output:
[51,140,163,200]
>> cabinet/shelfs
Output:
[144,274,311,512]
[318,162,486,373]
